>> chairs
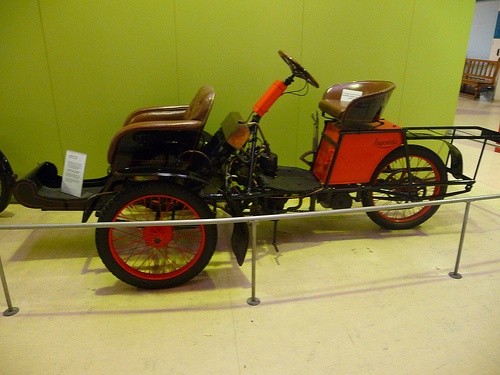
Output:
[107,85,216,165]
[320,80,396,124]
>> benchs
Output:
[460,57,499,101]
